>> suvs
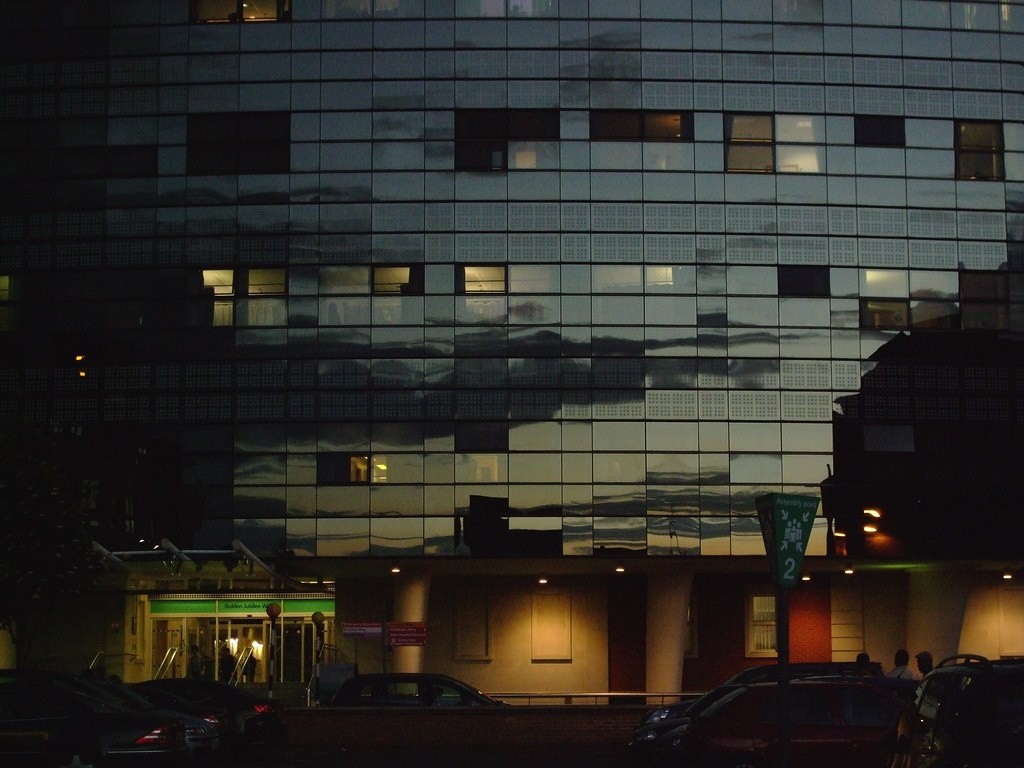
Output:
[884,652,1024,768]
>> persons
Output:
[915,651,933,677]
[189,645,257,683]
[856,653,870,663]
[886,649,917,679]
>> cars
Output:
[624,658,918,768]
[329,671,513,708]
[1,669,288,768]
